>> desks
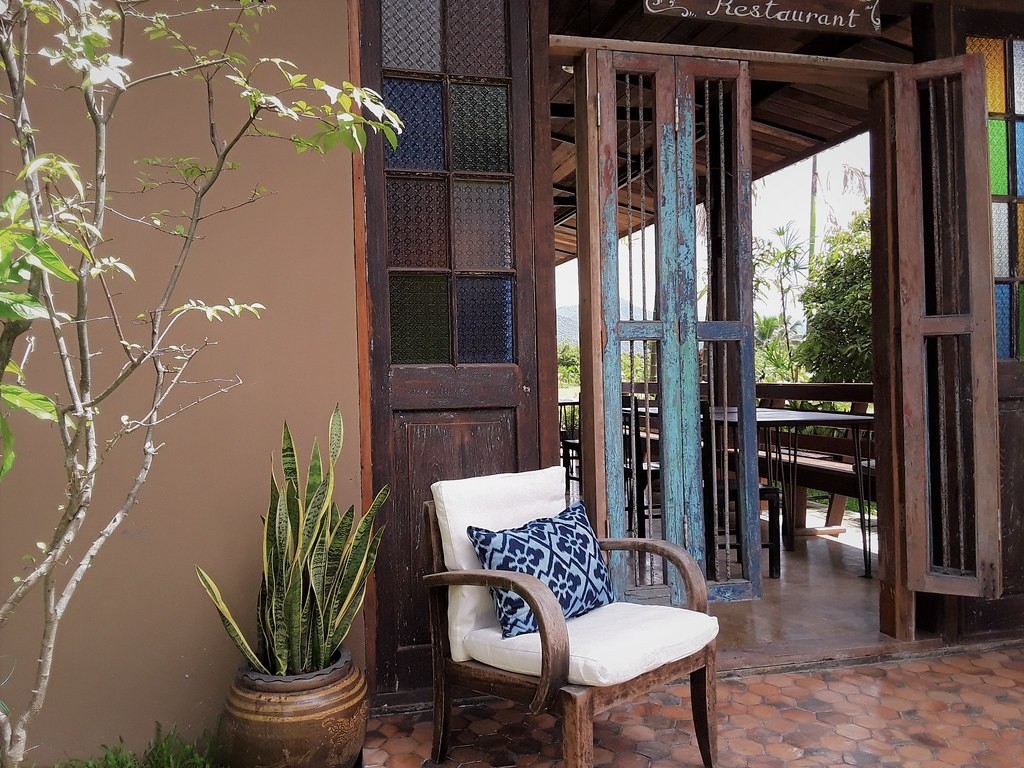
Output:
[624,405,875,580]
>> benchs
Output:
[556,379,877,579]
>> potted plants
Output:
[193,401,391,768]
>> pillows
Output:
[466,498,617,639]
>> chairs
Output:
[420,466,719,768]
